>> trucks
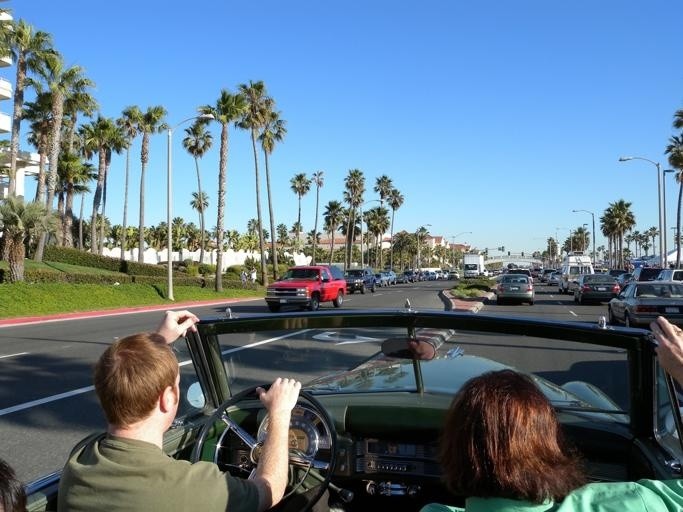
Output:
[556,250,595,294]
[462,252,485,278]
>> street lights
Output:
[360,198,385,269]
[572,209,596,271]
[583,223,588,228]
[166,112,217,300]
[616,155,665,269]
[416,223,432,269]
[663,169,675,269]
[452,231,473,266]
[556,227,572,251]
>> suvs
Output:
[262,263,347,312]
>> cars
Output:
[0,301,683,511]
[480,265,683,332]
[337,267,461,296]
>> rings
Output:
[674,329,682,336]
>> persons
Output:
[249,265,256,286]
[417,314,682,512]
[55,308,301,512]
[0,456,29,511]
[240,269,247,289]
